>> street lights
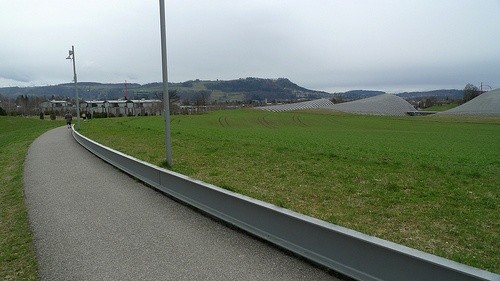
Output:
[67,45,81,129]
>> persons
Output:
[65,111,72,129]
